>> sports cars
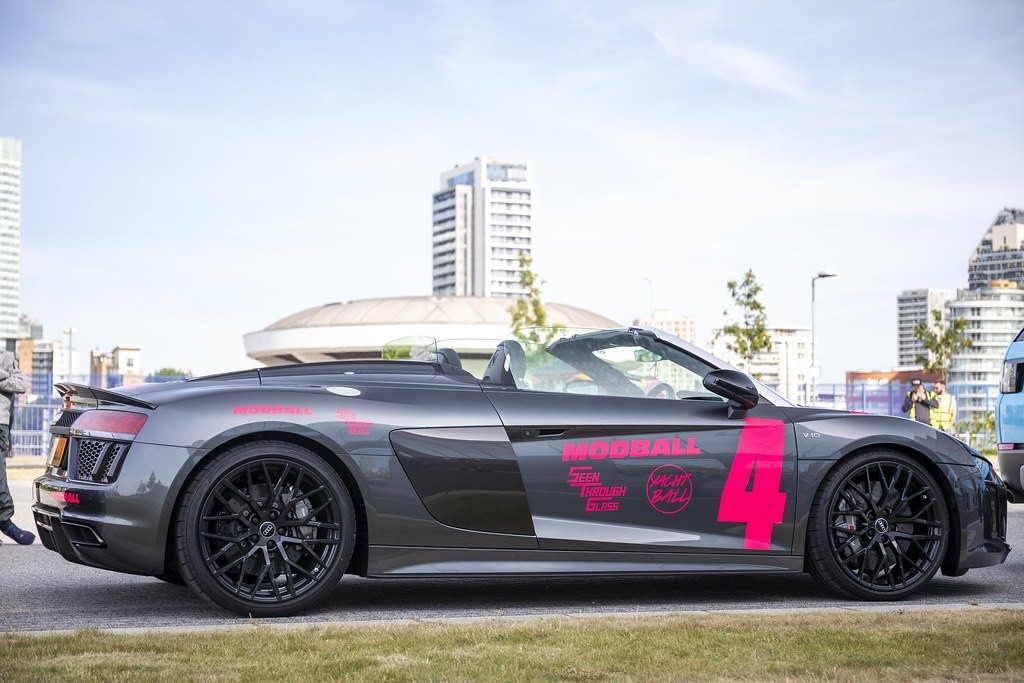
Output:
[31,324,1012,617]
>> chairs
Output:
[483,340,529,388]
[433,348,462,369]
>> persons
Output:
[902,377,939,426]
[0,349,36,545]
[930,381,957,438]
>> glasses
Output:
[933,387,939,390]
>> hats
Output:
[911,378,922,385]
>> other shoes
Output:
[1,523,35,545]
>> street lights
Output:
[811,271,836,410]
